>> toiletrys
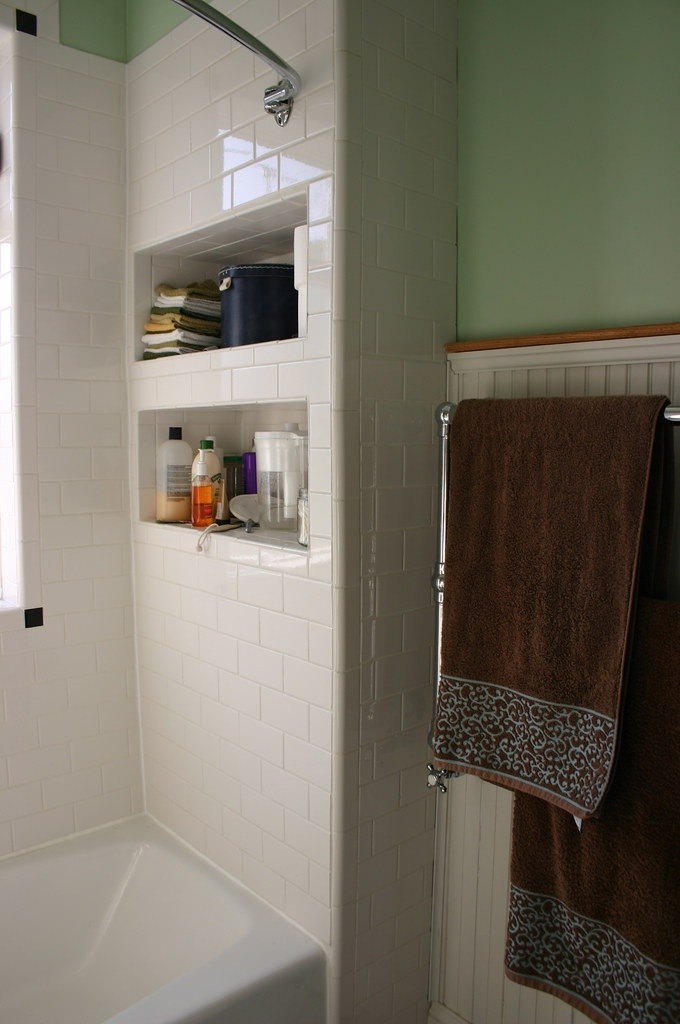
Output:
[154,421,308,546]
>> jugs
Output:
[255,431,306,529]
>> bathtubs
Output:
[1,812,328,1024]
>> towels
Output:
[139,278,221,361]
[429,395,680,1024]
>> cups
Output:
[223,455,244,519]
[296,486,308,547]
